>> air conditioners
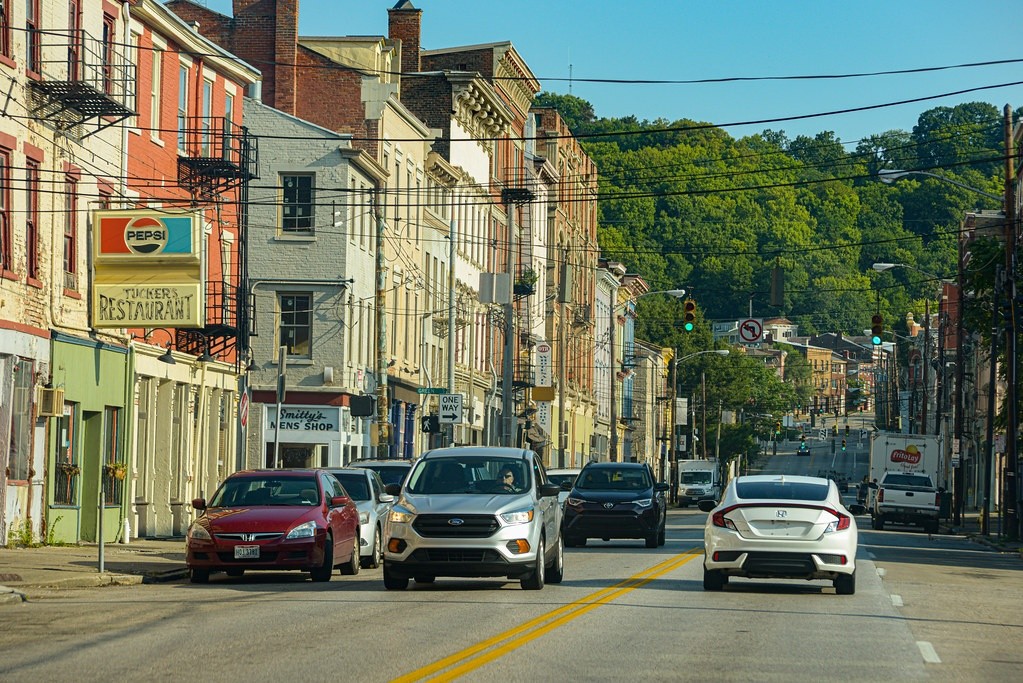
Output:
[38,387,64,417]
[13,388,28,416]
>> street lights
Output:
[872,221,966,526]
[671,347,729,500]
[879,102,1021,543]
[864,299,931,434]
[608,285,687,462]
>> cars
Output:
[561,458,669,549]
[318,456,415,568]
[798,445,811,456]
[187,467,362,583]
[383,445,566,590]
[546,468,581,509]
[697,472,859,596]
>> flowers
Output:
[61,463,80,477]
[105,461,128,480]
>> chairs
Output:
[297,489,318,502]
[245,488,272,502]
[593,472,609,483]
[436,465,468,491]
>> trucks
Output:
[870,431,947,494]
[676,458,719,508]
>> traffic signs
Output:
[439,394,463,425]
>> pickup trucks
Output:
[873,470,943,532]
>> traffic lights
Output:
[422,415,440,434]
[349,394,374,416]
[682,298,699,334]
[845,426,850,437]
[842,440,846,452]
[872,315,884,346]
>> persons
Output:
[857,475,868,500]
[496,467,522,493]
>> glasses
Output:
[499,474,511,479]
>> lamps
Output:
[166,331,214,362]
[131,328,175,364]
[216,345,261,371]
[390,360,396,366]
